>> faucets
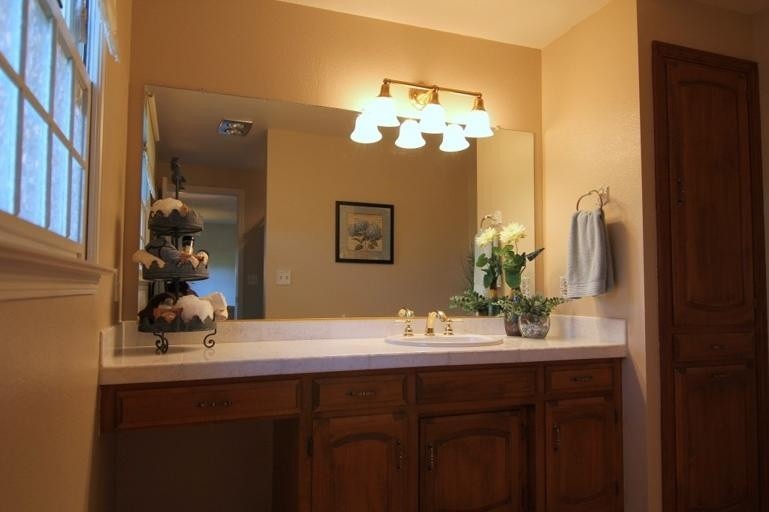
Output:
[425,311,449,335]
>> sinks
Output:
[384,334,504,348]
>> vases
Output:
[504,312,521,336]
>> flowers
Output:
[499,222,545,315]
[464,211,508,288]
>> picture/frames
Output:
[335,200,394,264]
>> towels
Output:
[565,208,614,298]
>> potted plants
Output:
[446,290,508,316]
[491,291,574,338]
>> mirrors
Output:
[137,82,535,320]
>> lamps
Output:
[350,112,470,152]
[369,77,495,138]
